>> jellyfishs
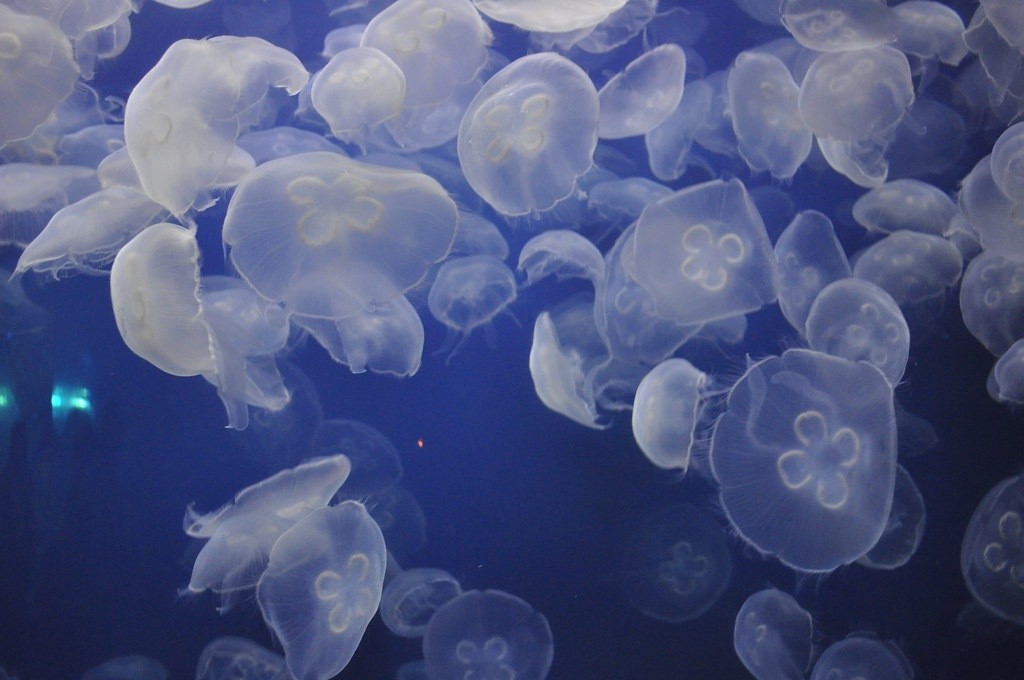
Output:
[0,1,1023,680]
